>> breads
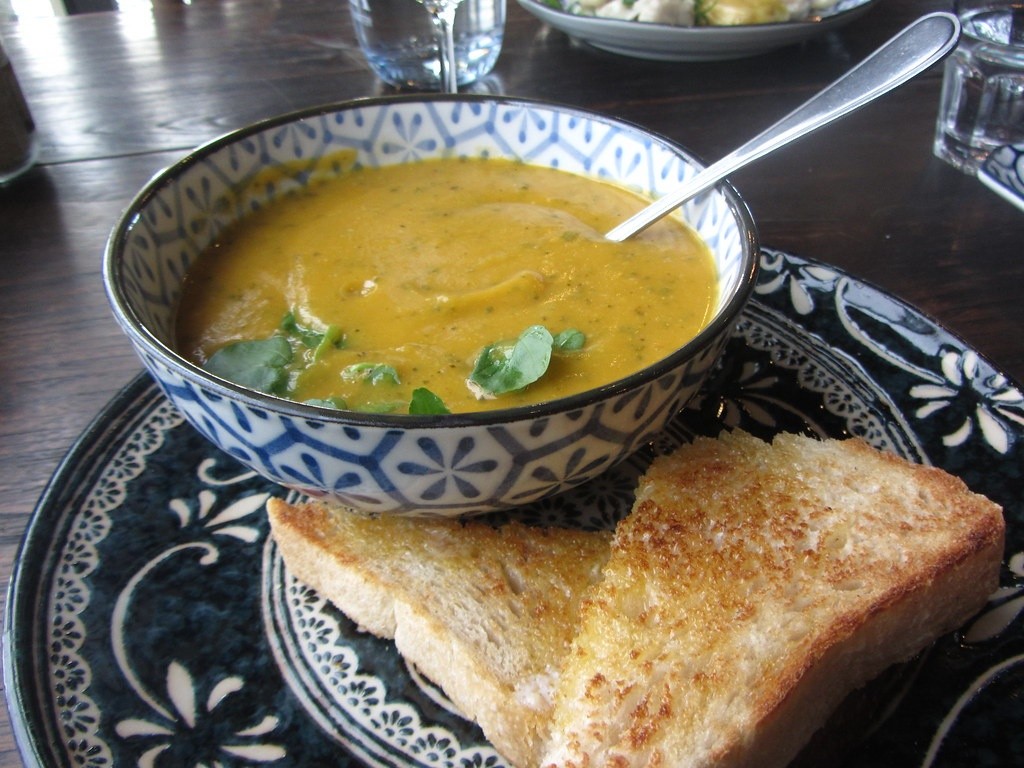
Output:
[537,428,1006,767]
[268,494,615,768]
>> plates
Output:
[514,0,876,62]
[2,249,1024,768]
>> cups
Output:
[346,0,507,89]
[0,45,42,183]
[934,6,1024,177]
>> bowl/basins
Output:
[100,91,763,520]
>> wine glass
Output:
[418,0,459,94]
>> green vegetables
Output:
[199,310,597,418]
